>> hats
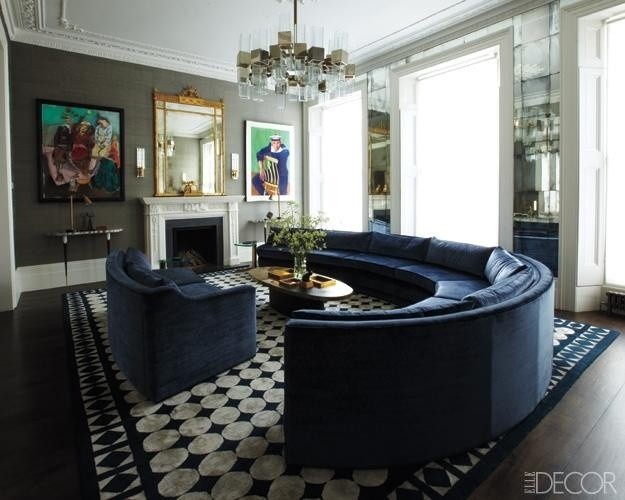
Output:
[269,134,282,141]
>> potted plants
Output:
[268,204,327,279]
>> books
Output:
[267,269,295,281]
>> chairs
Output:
[261,155,291,195]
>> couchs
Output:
[101,246,259,405]
[256,217,561,470]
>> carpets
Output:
[61,264,622,500]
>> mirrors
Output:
[146,84,226,196]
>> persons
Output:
[91,116,121,194]
[251,134,291,196]
[70,120,95,180]
[110,133,121,170]
[51,125,72,182]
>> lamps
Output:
[230,154,240,181]
[235,0,359,105]
[137,146,147,178]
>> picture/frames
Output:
[35,96,128,207]
[245,119,296,203]
